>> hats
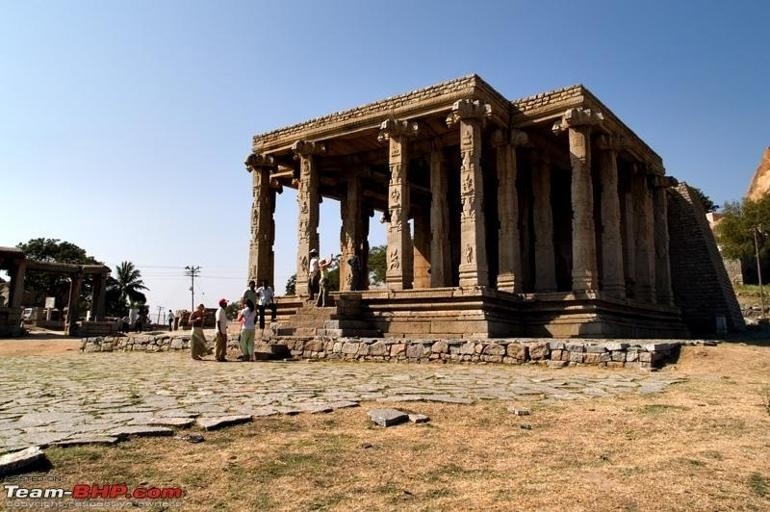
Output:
[219,299,228,305]
[309,249,317,253]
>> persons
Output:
[256,279,277,332]
[213,299,228,362]
[168,309,174,331]
[306,248,320,301]
[135,310,143,334]
[243,280,259,325]
[314,253,333,307]
[188,304,210,360]
[237,300,256,361]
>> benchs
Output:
[73,321,113,338]
